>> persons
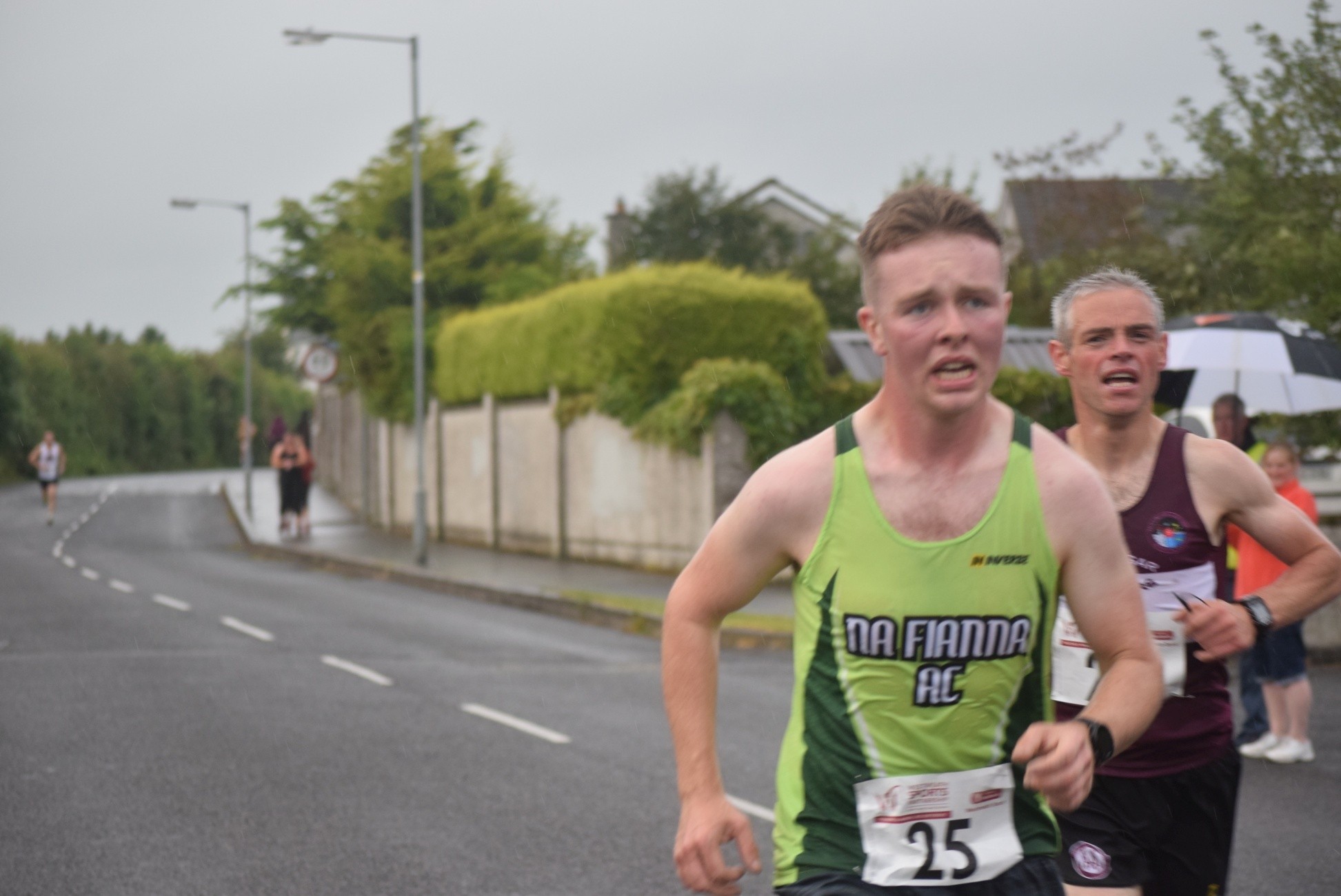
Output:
[237,414,258,467]
[1206,393,1270,746]
[270,428,317,543]
[1052,269,1341,896]
[27,427,68,523]
[1225,440,1315,765]
[659,178,1165,895]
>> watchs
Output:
[1076,717,1114,767]
[1232,594,1273,646]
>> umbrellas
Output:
[1150,312,1341,455]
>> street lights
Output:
[286,29,432,563]
[171,198,252,523]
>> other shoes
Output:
[1239,731,1280,760]
[1265,738,1315,764]
[1233,727,1270,746]
